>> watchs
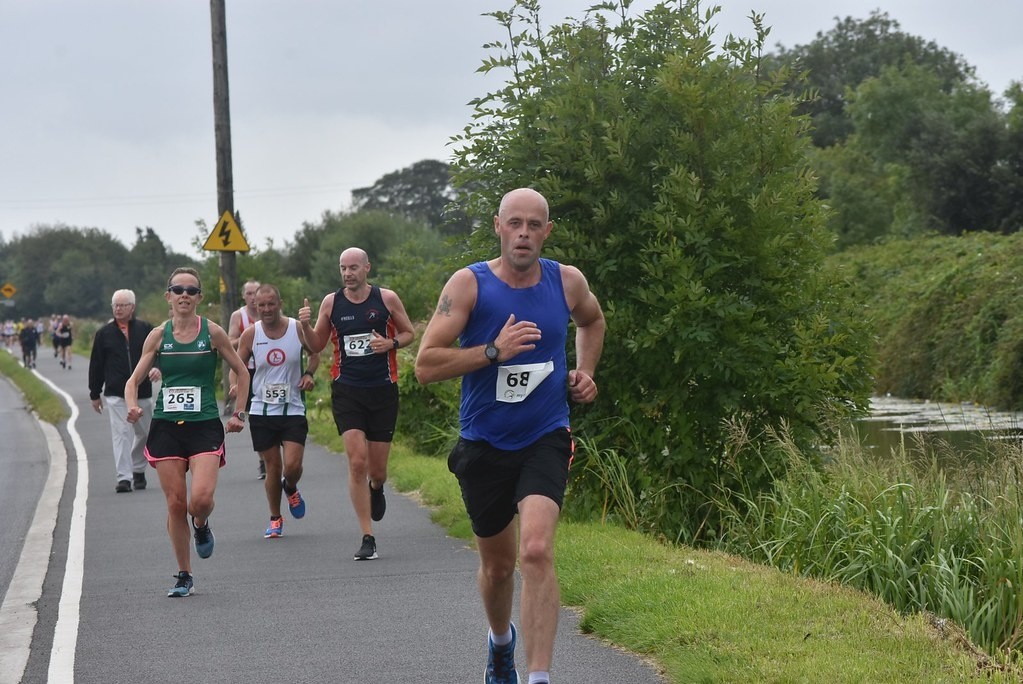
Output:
[484,340,502,367]
[233,411,246,421]
[391,338,399,349]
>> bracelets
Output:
[305,370,314,378]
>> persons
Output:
[227,280,285,480]
[0,312,74,371]
[298,246,415,560]
[88,289,161,493]
[124,268,251,597]
[415,189,606,684]
[229,283,319,538]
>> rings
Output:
[375,346,377,349]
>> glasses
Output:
[111,304,130,309]
[167,287,201,296]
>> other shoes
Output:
[133,472,147,490]
[115,480,132,492]
[24,365,31,368]
[33,363,36,369]
[60,361,65,368]
[68,366,71,369]
[55,353,57,357]
[257,475,266,480]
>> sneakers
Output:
[484,622,521,684]
[353,534,379,561]
[263,516,283,539]
[192,516,215,560]
[369,480,386,522]
[167,571,195,597]
[282,477,305,519]
